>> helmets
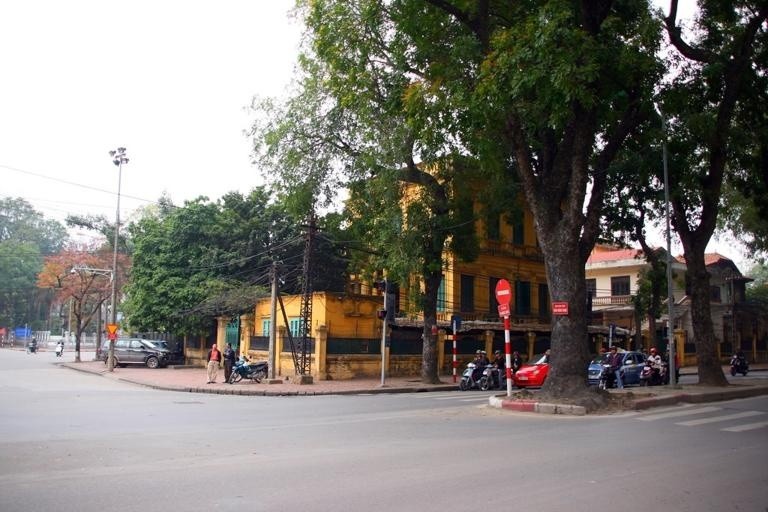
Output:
[600,348,606,352]
[650,348,657,353]
[495,350,502,354]
[475,350,487,355]
[609,346,617,351]
[513,351,519,356]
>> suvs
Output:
[97,338,169,369]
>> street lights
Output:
[107,146,130,372]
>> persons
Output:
[488,349,506,391]
[206,344,222,383]
[479,350,490,370]
[60,342,64,354]
[511,350,522,374]
[222,343,235,383]
[233,344,240,361]
[468,349,484,383]
[597,344,684,390]
[54,341,64,357]
[28,336,37,354]
[732,346,745,375]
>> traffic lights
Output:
[372,280,386,293]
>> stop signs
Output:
[494,278,513,305]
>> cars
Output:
[588,350,648,385]
[515,350,552,387]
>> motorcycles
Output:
[598,363,625,391]
[459,362,515,391]
[228,352,269,385]
[730,354,749,376]
[638,355,669,387]
